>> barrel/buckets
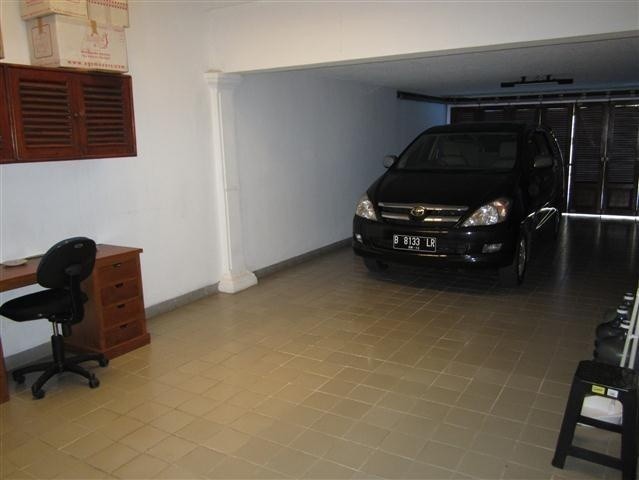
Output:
[593,292,639,368]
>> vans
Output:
[351,122,565,290]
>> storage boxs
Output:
[20,0,90,22]
[25,14,129,75]
[89,0,131,27]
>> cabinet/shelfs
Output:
[0,61,137,168]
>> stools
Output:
[548,359,637,480]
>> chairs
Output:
[0,237,110,399]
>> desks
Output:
[1,244,152,405]
